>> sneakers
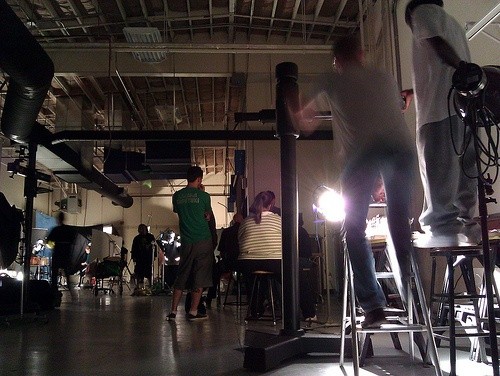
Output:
[166,313,176,321]
[459,229,481,247]
[305,315,317,320]
[361,309,386,329]
[187,311,209,322]
[414,232,457,247]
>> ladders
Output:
[335,237,446,376]
[424,250,500,376]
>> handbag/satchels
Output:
[151,285,171,296]
[132,287,149,296]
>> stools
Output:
[222,264,312,327]
[429,245,499,376]
[95,275,115,295]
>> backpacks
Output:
[185,292,206,314]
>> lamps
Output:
[446,64,500,376]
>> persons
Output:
[81,246,97,287]
[401,0,485,250]
[121,241,128,285]
[217,190,317,323]
[130,224,156,296]
[165,165,218,322]
[289,42,420,328]
[185,182,217,313]
[32,211,81,306]
